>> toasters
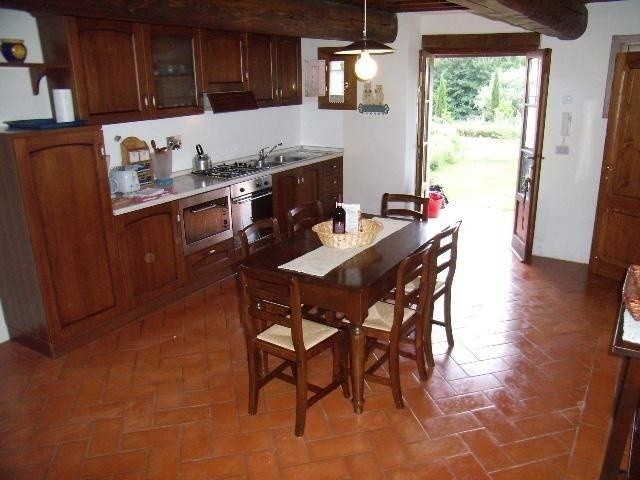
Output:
[108,166,140,195]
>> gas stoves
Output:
[191,161,262,179]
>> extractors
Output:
[203,89,260,115]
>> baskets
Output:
[312,218,384,249]
[622,264,640,321]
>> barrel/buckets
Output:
[427,193,444,218]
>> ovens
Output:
[179,187,234,256]
[230,175,274,250]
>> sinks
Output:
[244,159,286,170]
[265,153,308,164]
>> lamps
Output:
[333,1,396,81]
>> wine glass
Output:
[149,150,173,187]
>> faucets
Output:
[258,142,282,160]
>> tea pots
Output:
[191,144,212,170]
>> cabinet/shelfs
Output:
[70,16,205,124]
[274,157,350,235]
[247,33,303,106]
[199,30,246,92]
[114,200,234,312]
[13,130,124,349]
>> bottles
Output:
[0,38,28,63]
[333,191,346,234]
